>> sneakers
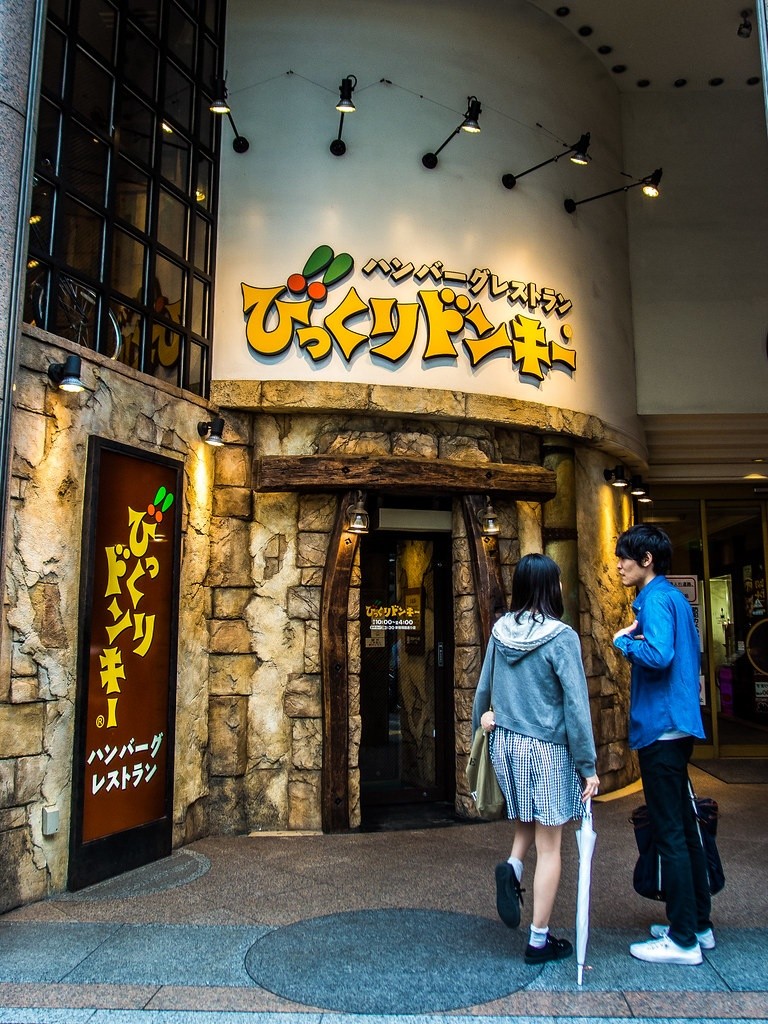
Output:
[494,862,525,930]
[524,933,573,964]
[651,923,716,949]
[630,934,703,966]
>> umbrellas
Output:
[576,796,597,984]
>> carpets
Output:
[689,758,768,784]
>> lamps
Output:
[197,418,224,446]
[422,96,482,169]
[48,355,85,392]
[502,131,593,189]
[481,495,501,535]
[737,10,753,38]
[209,78,249,153]
[564,168,663,214]
[347,490,370,534]
[330,74,357,156]
[604,464,652,503]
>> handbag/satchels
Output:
[465,726,506,820]
[630,797,726,902]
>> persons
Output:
[468,554,598,964]
[612,523,714,967]
